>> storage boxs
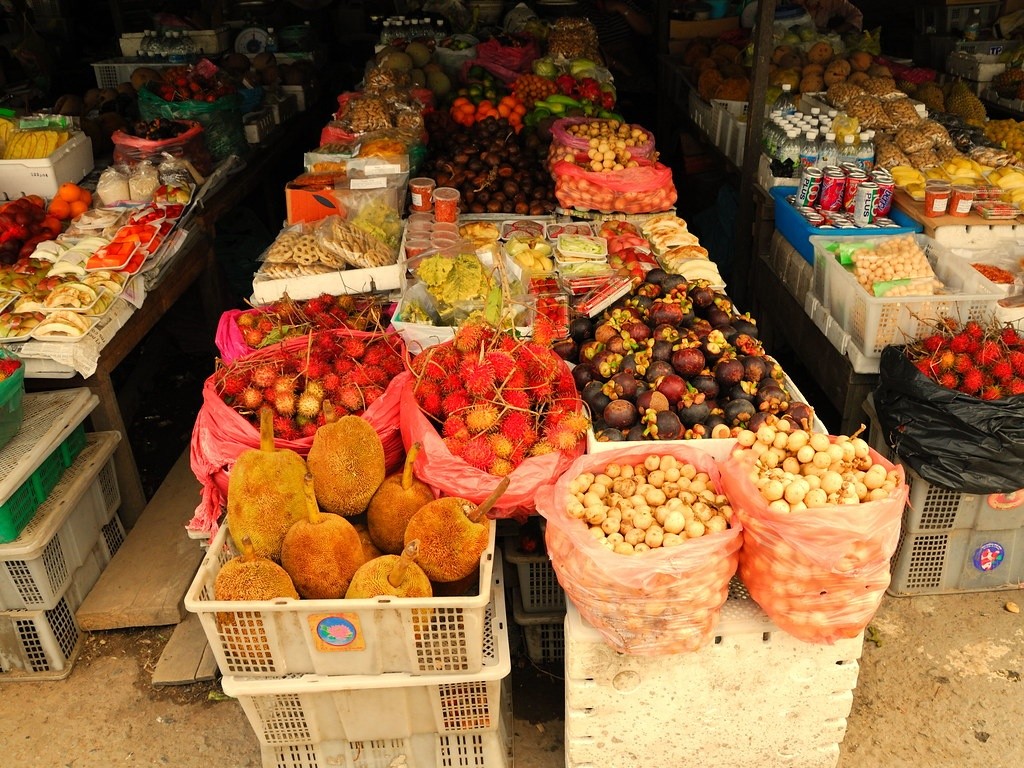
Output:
[0,1,1024,768]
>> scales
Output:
[234,2,276,59]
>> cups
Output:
[949,185,977,217]
[404,178,460,267]
[924,187,951,217]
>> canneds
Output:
[788,162,902,230]
[404,178,460,267]
[924,179,976,218]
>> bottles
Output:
[170,32,187,63]
[963,8,980,41]
[181,30,195,61]
[265,28,278,53]
[147,31,160,50]
[380,17,446,45]
[136,51,170,63]
[772,84,797,115]
[140,30,151,50]
[767,117,876,177]
[161,32,173,50]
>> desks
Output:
[758,254,879,440]
[24,232,223,527]
[75,121,303,314]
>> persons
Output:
[587,0,653,132]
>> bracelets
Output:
[612,59,616,67]
[624,7,632,17]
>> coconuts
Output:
[381,43,450,98]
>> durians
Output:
[899,73,988,121]
[683,27,753,103]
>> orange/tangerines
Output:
[46,182,91,219]
[450,96,526,134]
